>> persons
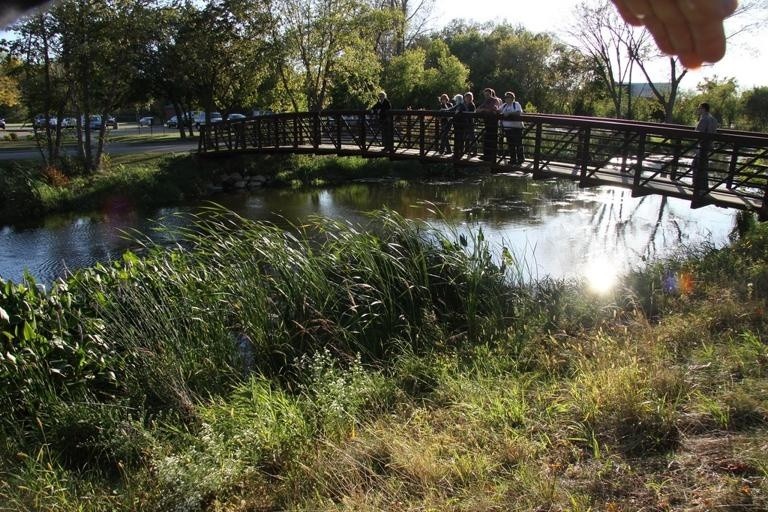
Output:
[608,0,737,70]
[371,91,392,152]
[690,100,718,190]
[493,90,525,165]
[475,87,501,163]
[441,93,465,159]
[458,91,477,159]
[437,92,453,154]
[491,88,503,112]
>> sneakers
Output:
[439,147,525,165]
[381,147,395,153]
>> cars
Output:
[33,115,116,131]
[141,117,163,126]
[167,112,246,131]
